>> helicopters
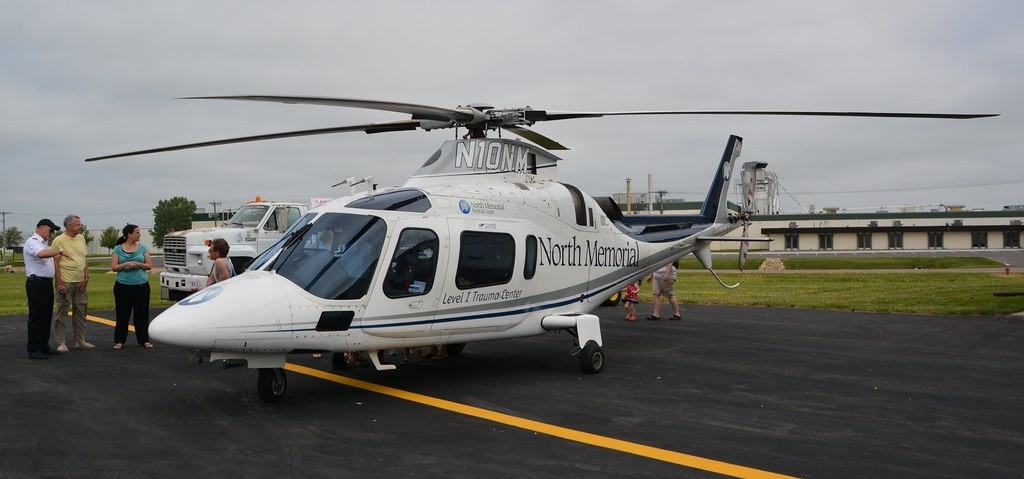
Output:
[86,90,1003,402]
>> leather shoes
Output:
[42,350,61,355]
[27,349,49,360]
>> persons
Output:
[203,238,236,287]
[23,218,77,360]
[311,222,335,251]
[343,341,447,366]
[52,214,97,353]
[646,260,682,321]
[620,282,640,321]
[111,224,154,349]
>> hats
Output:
[37,218,61,231]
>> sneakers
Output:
[56,343,69,352]
[389,349,400,356]
[73,340,96,350]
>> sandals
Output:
[422,350,439,357]
[145,342,154,348]
[431,353,448,360]
[112,342,123,349]
[401,355,410,364]
[346,359,370,367]
[417,357,427,367]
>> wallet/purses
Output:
[124,265,141,272]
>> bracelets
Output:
[84,275,90,279]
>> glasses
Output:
[48,229,55,233]
[209,249,218,252]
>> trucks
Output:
[162,202,323,302]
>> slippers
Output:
[646,315,660,320]
[669,314,681,321]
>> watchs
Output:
[58,248,64,255]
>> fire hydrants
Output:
[1005,265,1010,274]
